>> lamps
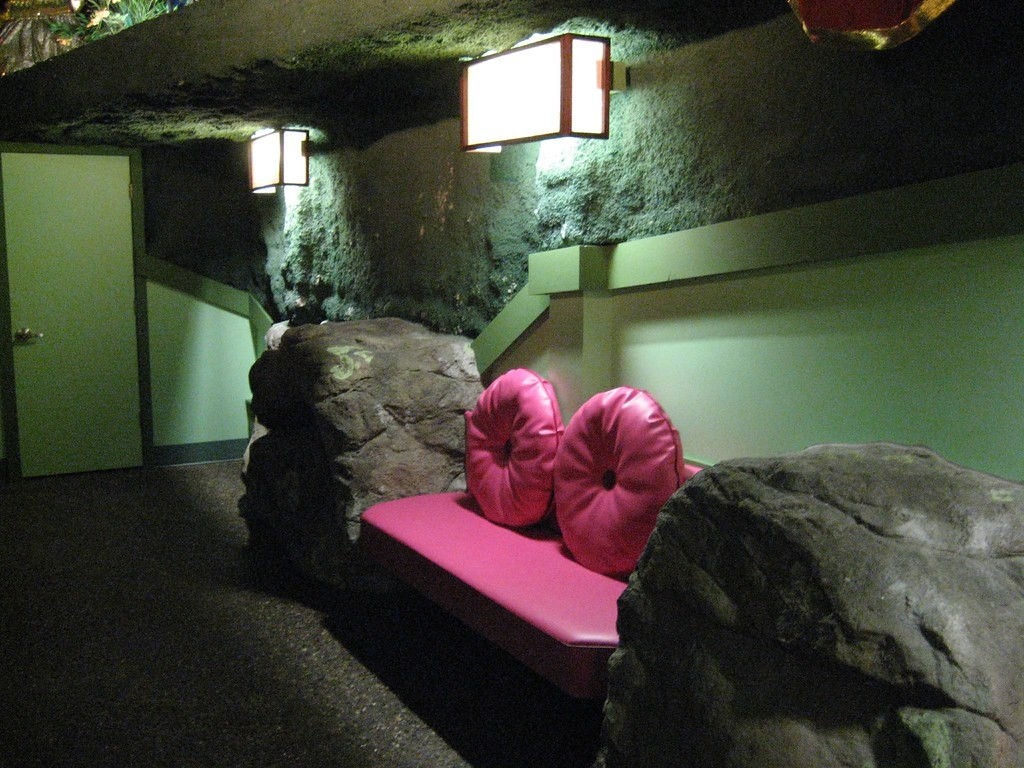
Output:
[459,32,630,153]
[247,128,317,194]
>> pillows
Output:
[460,368,565,528]
[551,384,681,578]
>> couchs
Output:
[351,365,710,698]
